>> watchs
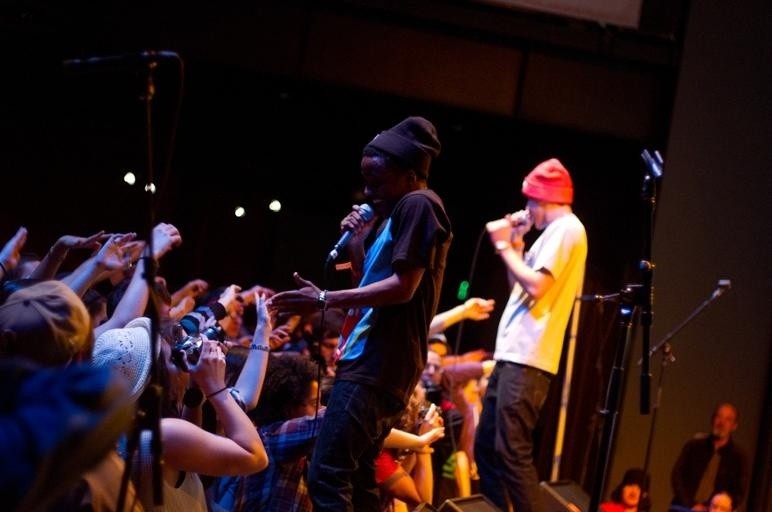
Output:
[495,240,512,254]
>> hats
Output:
[368,116,441,179]
[91,317,163,408]
[521,158,576,205]
[0,279,89,362]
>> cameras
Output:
[171,326,223,372]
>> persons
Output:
[598,467,652,512]
[473,160,588,511]
[264,119,453,512]
[0,223,495,512]
[708,492,738,512]
[667,403,742,512]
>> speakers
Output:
[416,479,593,512]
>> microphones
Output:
[324,204,372,261]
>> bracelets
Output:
[513,240,525,249]
